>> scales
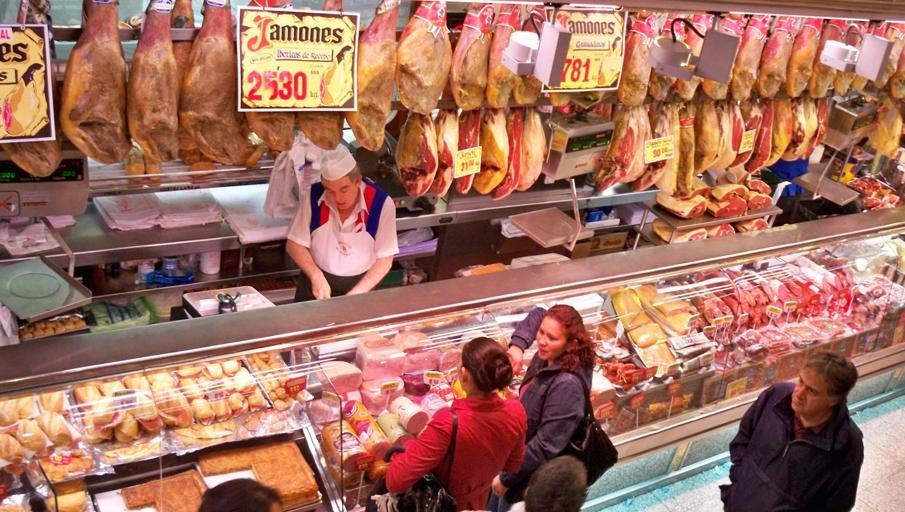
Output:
[0,141,93,323]
[794,96,878,205]
[508,111,617,251]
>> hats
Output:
[321,143,357,180]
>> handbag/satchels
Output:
[366,472,457,512]
[560,416,618,487]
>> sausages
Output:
[672,254,853,333]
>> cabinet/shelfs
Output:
[0,205,905,510]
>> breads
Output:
[0,353,320,512]
[19,316,86,339]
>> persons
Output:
[524,453,590,510]
[194,478,284,512]
[719,348,867,512]
[386,335,528,512]
[485,302,594,511]
[283,144,401,301]
